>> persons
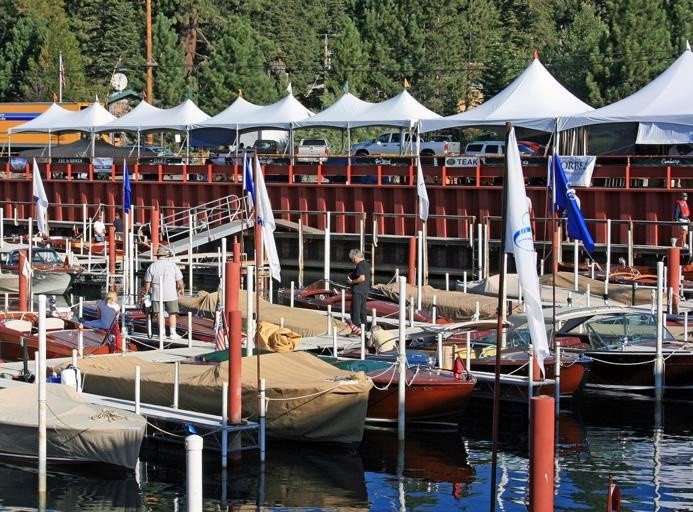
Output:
[84,292,121,330]
[112,213,123,232]
[143,245,185,340]
[236,143,247,164]
[344,248,371,331]
[224,145,233,165]
[94,215,106,242]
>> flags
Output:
[32,159,48,236]
[505,126,550,379]
[415,144,430,222]
[550,146,594,262]
[123,160,132,214]
[244,156,282,283]
[213,293,226,352]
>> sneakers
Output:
[170,333,183,340]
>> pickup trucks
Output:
[351,131,460,164]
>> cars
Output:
[463,141,545,166]
[124,144,182,164]
[427,135,457,142]
[252,139,288,164]
[294,137,330,165]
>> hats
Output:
[154,247,170,256]
